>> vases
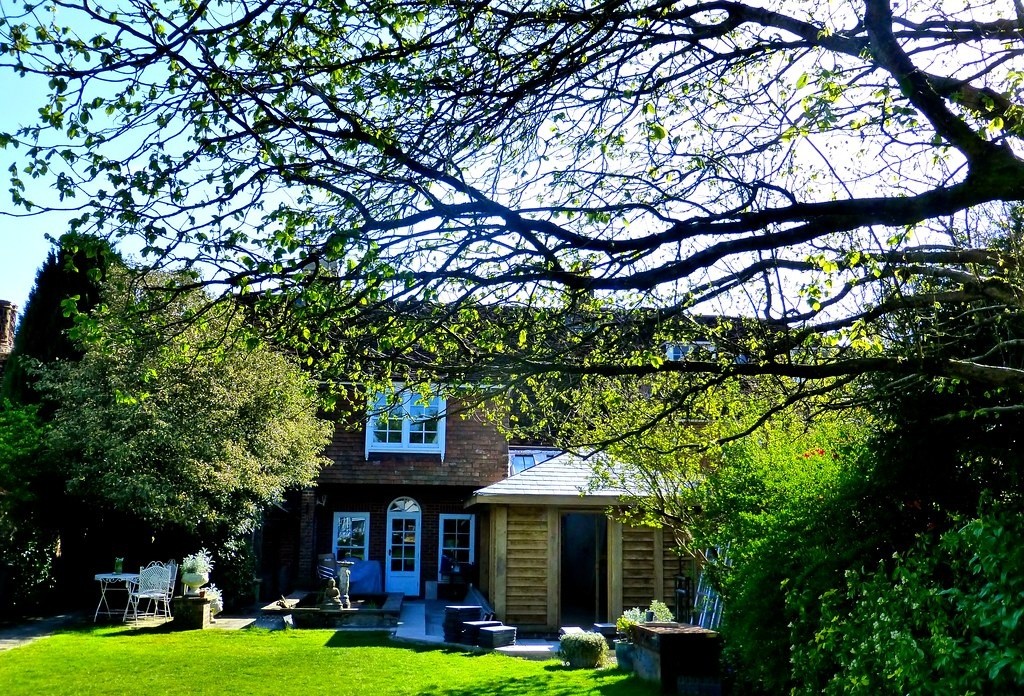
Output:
[115,561,122,574]
[181,572,209,595]
[209,603,223,623]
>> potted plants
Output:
[555,631,613,668]
[613,600,675,672]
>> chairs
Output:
[133,559,178,620]
[318,554,340,590]
[123,561,171,625]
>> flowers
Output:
[180,547,215,572]
[116,558,124,561]
[200,584,223,606]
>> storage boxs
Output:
[443,606,516,648]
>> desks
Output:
[94,573,140,623]
